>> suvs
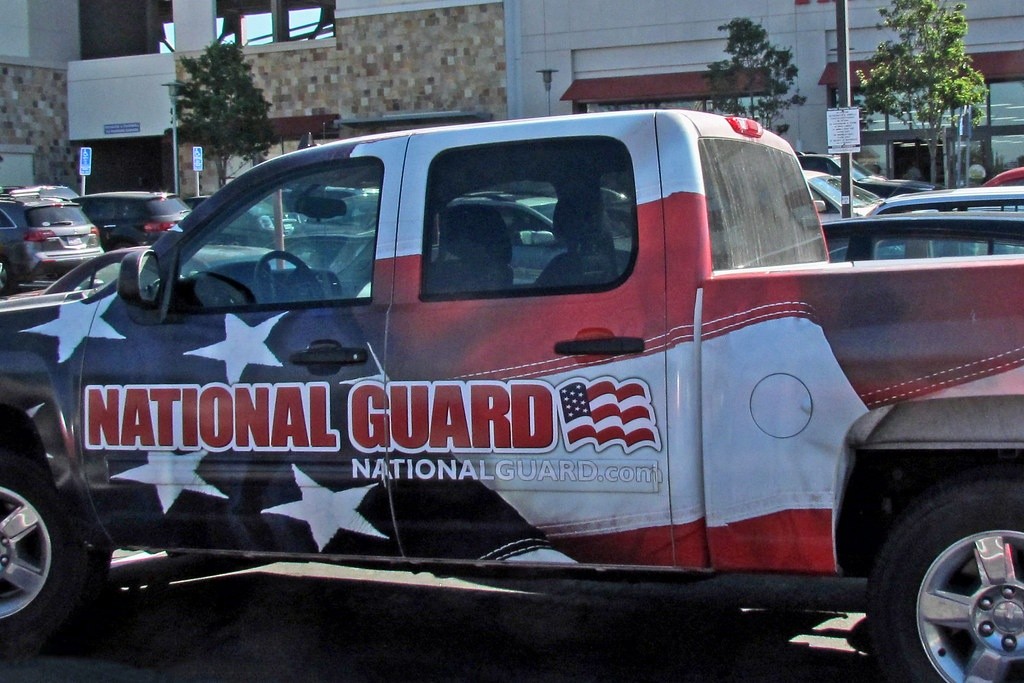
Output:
[0,195,105,297]
[794,151,936,199]
[446,189,558,268]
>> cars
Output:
[9,184,79,208]
[821,212,1024,263]
[983,167,1024,185]
[801,170,882,223]
[183,196,297,247]
[70,191,192,250]
[263,187,379,226]
[863,186,1024,260]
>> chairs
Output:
[537,178,629,288]
[427,206,514,291]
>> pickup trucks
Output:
[0,109,1024,683]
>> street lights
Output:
[161,81,185,194]
